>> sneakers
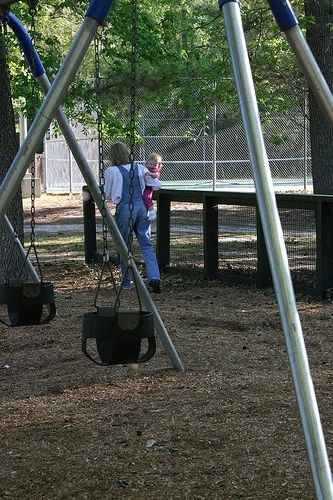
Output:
[148,213,157,220]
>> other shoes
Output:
[122,286,131,289]
[151,279,161,294]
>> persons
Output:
[103,142,161,293]
[141,152,163,221]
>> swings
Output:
[80,0,157,366]
[2,1,58,329]
